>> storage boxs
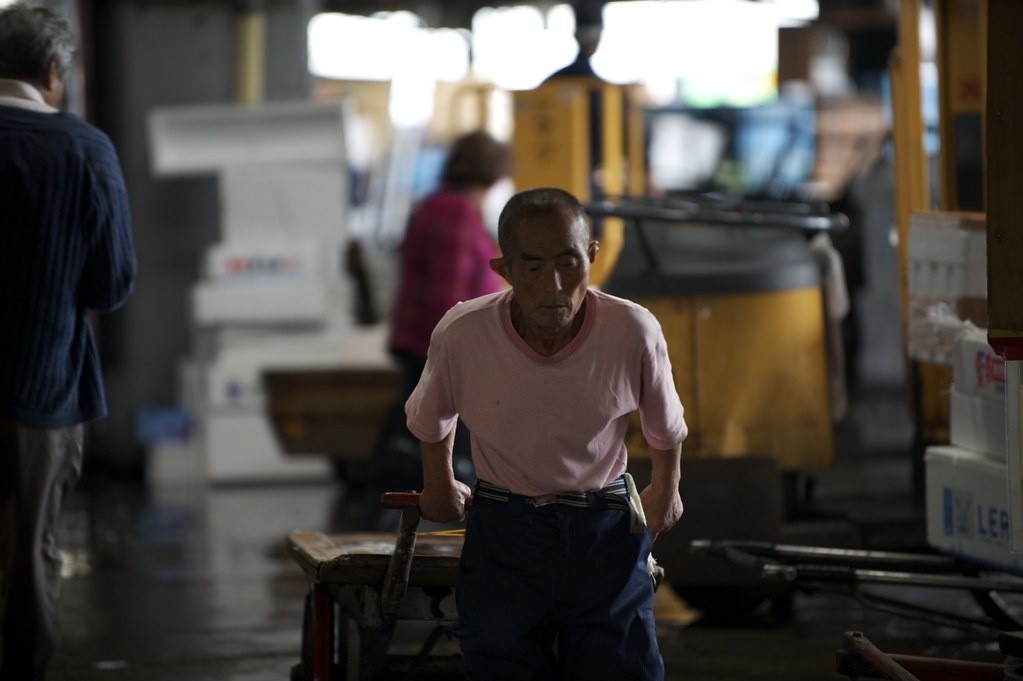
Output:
[190,273,334,330]
[216,161,342,244]
[923,445,1023,570]
[949,383,1005,462]
[200,244,350,326]
[903,209,987,362]
[955,334,1005,402]
[201,484,333,578]
[204,328,395,416]
[145,96,345,175]
[204,410,334,487]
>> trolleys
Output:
[299,491,668,681]
[684,537,1023,681]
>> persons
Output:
[402,187,692,681]
[0,6,137,681]
[390,128,508,444]
